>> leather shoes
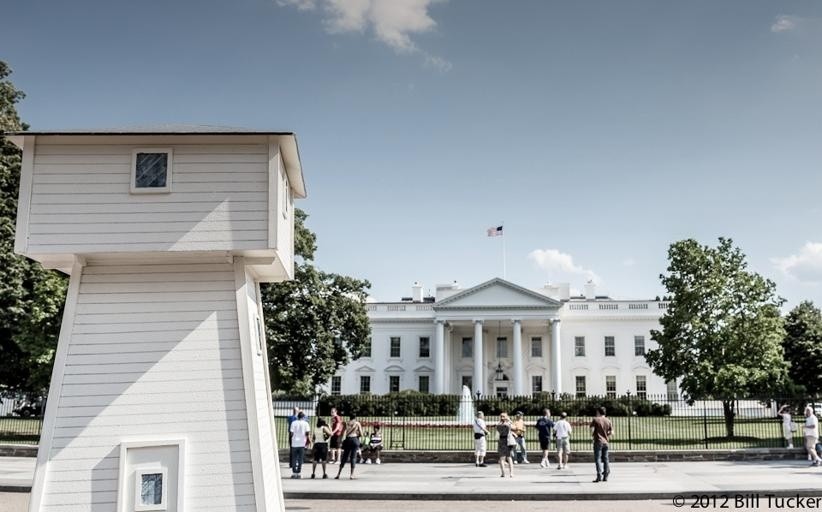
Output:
[593,478,601,483]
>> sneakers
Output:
[522,460,530,464]
[376,458,381,464]
[365,459,371,464]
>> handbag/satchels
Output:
[474,433,483,440]
[507,424,517,447]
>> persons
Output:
[310,407,371,480]
[816,436,822,459]
[287,406,309,468]
[472,411,491,468]
[590,406,613,483]
[535,408,556,468]
[553,412,572,470]
[497,412,517,478]
[777,403,796,451]
[365,424,383,465]
[289,411,310,479]
[511,411,530,465]
[801,406,822,467]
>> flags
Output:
[486,225,504,239]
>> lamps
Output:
[495,320,504,380]
